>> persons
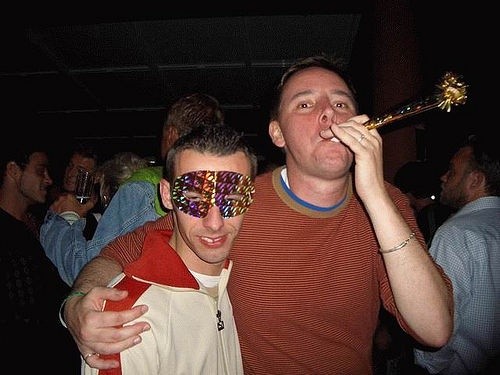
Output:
[374,136,500,375]
[79,125,260,375]
[39,94,223,287]
[87,152,147,231]
[0,138,81,375]
[61,55,454,375]
[60,143,101,192]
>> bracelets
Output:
[379,233,417,253]
[59,290,85,329]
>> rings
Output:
[84,351,99,359]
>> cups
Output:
[75,172,94,204]
